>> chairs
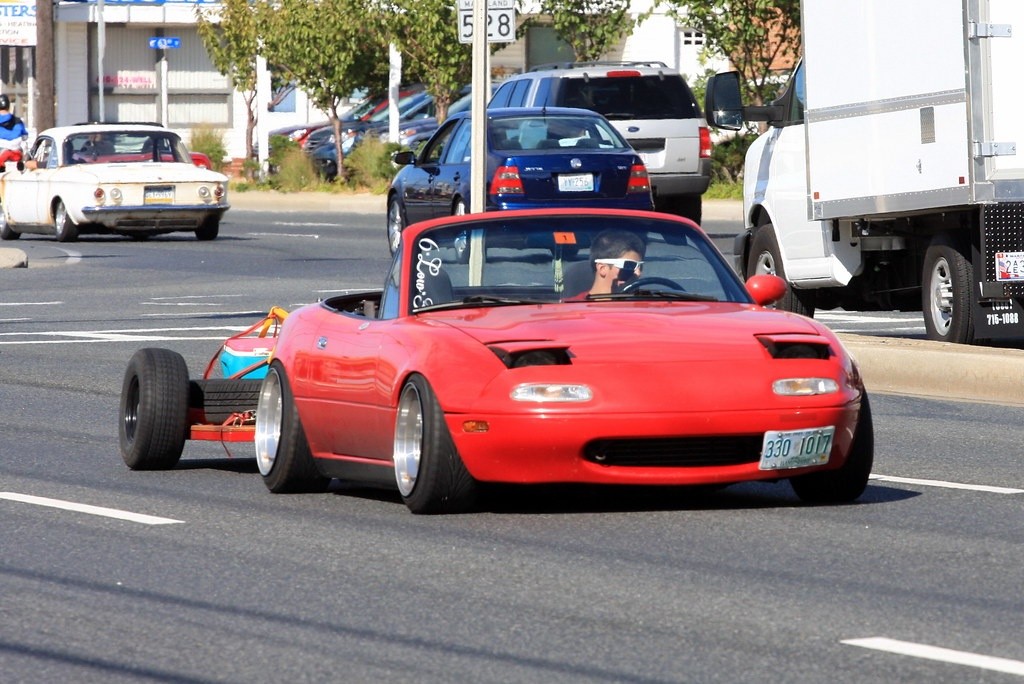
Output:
[141,139,165,153]
[563,260,595,299]
[496,140,521,150]
[537,140,560,149]
[577,138,599,148]
[412,262,452,311]
[81,140,116,154]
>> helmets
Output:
[0,94,10,108]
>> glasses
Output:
[595,259,645,272]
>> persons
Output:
[559,229,646,300]
[83,133,116,154]
[0,94,28,173]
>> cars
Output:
[387,107,653,256]
[252,80,509,187]
[0,122,231,243]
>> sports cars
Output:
[257,207,874,516]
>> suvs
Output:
[490,62,712,229]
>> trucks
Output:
[705,0,1024,342]
